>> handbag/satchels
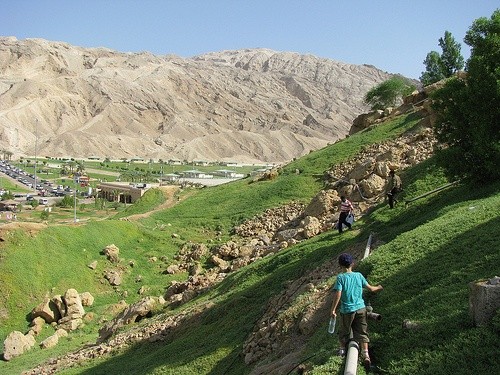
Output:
[346,215,354,224]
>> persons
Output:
[330,255,383,372]
[388,171,402,209]
[338,195,353,234]
[6,214,16,221]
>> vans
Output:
[39,198,48,205]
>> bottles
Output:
[329,317,336,333]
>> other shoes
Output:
[336,350,346,357]
[363,356,371,367]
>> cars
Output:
[0,160,38,186]
[37,178,72,196]
[26,196,34,201]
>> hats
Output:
[337,254,354,267]
